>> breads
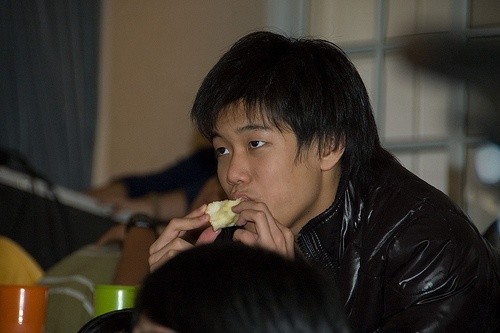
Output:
[205,198,242,231]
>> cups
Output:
[0,285,48,333]
[94,285,138,316]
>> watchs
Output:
[126,213,156,233]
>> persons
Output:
[148,29,500,333]
[130,241,351,333]
[90,145,224,287]
